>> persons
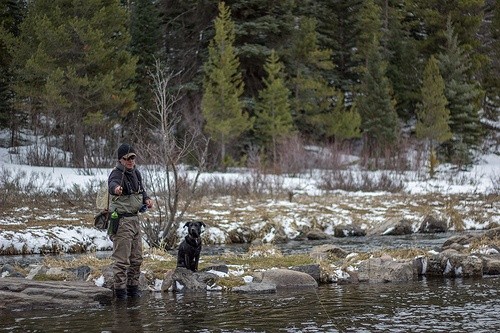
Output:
[107,143,154,300]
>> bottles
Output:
[107,208,118,235]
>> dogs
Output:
[176,219,207,274]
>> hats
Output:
[118,144,137,161]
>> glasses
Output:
[120,155,136,160]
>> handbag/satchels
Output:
[94,207,110,229]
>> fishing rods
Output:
[111,107,142,202]
